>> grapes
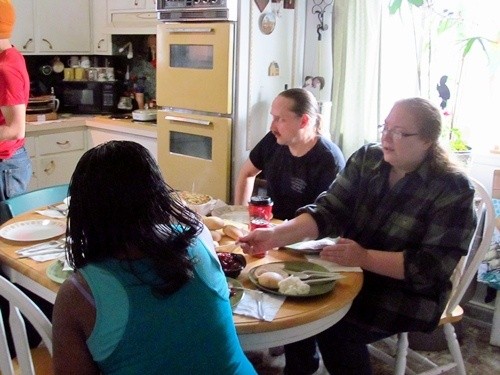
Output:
[215,252,240,270]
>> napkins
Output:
[15,240,70,262]
[232,288,286,321]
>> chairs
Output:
[0,277,55,375]
[367,176,495,375]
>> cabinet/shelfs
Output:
[9,1,159,56]
[22,127,157,195]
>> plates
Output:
[283,236,343,254]
[225,277,245,306]
[46,257,72,284]
[26,96,51,113]
[247,260,336,297]
[0,219,69,241]
[211,204,249,226]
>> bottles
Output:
[248,197,273,259]
[121,45,156,110]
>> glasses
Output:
[377,123,420,140]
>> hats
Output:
[0,0,17,39]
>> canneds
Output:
[250,219,270,258]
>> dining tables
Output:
[0,192,365,351]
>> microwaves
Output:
[52,78,121,117]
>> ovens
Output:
[153,19,239,204]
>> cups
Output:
[50,95,60,114]
[39,56,114,82]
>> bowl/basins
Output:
[214,228,249,253]
[217,252,246,279]
[184,193,217,217]
[132,108,156,121]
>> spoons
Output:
[293,273,342,279]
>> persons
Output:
[239,98,478,375]
[0,0,32,225]
[233,88,348,357]
[51,140,259,375]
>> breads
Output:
[258,272,309,295]
[203,217,243,247]
